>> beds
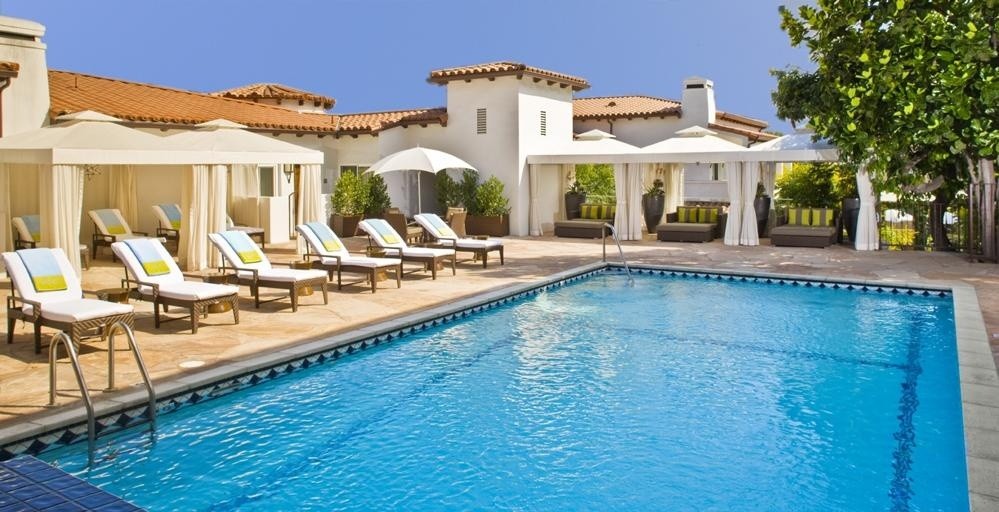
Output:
[554,203,616,239]
[655,200,730,242]
[770,207,840,247]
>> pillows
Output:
[581,205,615,219]
[788,207,834,226]
[676,206,718,223]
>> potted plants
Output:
[564,179,587,220]
[754,181,771,238]
[330,167,394,238]
[643,178,666,234]
[843,189,860,242]
[431,167,513,237]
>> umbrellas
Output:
[360,143,479,214]
